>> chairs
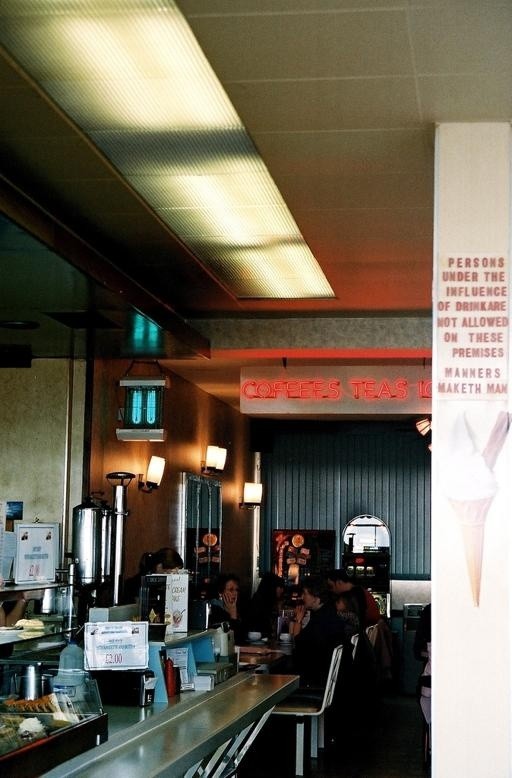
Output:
[268,623,378,775]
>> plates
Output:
[0,626,25,637]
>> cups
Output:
[174,614,183,627]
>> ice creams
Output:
[434,411,511,608]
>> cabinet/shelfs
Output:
[0,563,76,647]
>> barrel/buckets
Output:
[59,642,85,668]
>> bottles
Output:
[163,659,181,697]
[21,663,42,700]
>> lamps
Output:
[138,455,166,494]
[200,446,227,477]
[239,482,263,511]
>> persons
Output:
[0,573,29,629]
[259,569,382,703]
[205,568,287,654]
[119,547,183,607]
[413,604,430,727]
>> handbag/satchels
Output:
[336,589,392,675]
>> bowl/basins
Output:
[280,633,291,641]
[248,631,261,639]
